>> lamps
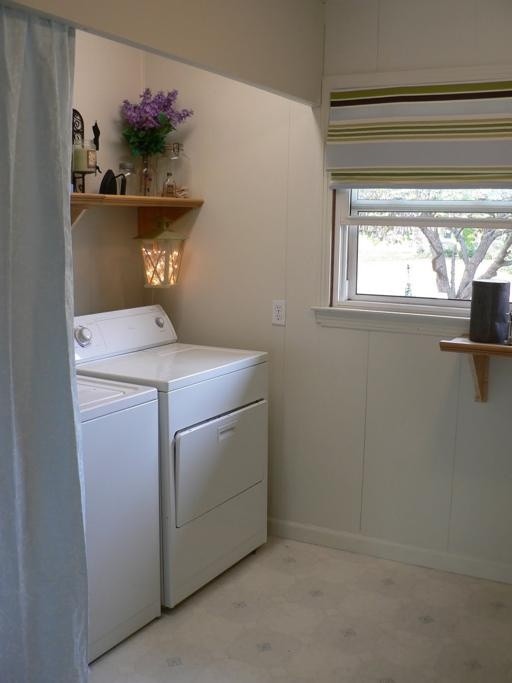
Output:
[129,212,192,291]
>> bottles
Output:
[161,171,176,197]
[117,160,138,196]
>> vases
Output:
[137,153,159,197]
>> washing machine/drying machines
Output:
[73,305,269,608]
[75,374,160,666]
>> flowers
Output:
[116,84,196,159]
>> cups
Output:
[470,275,512,343]
[72,138,97,173]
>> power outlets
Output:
[270,297,287,327]
